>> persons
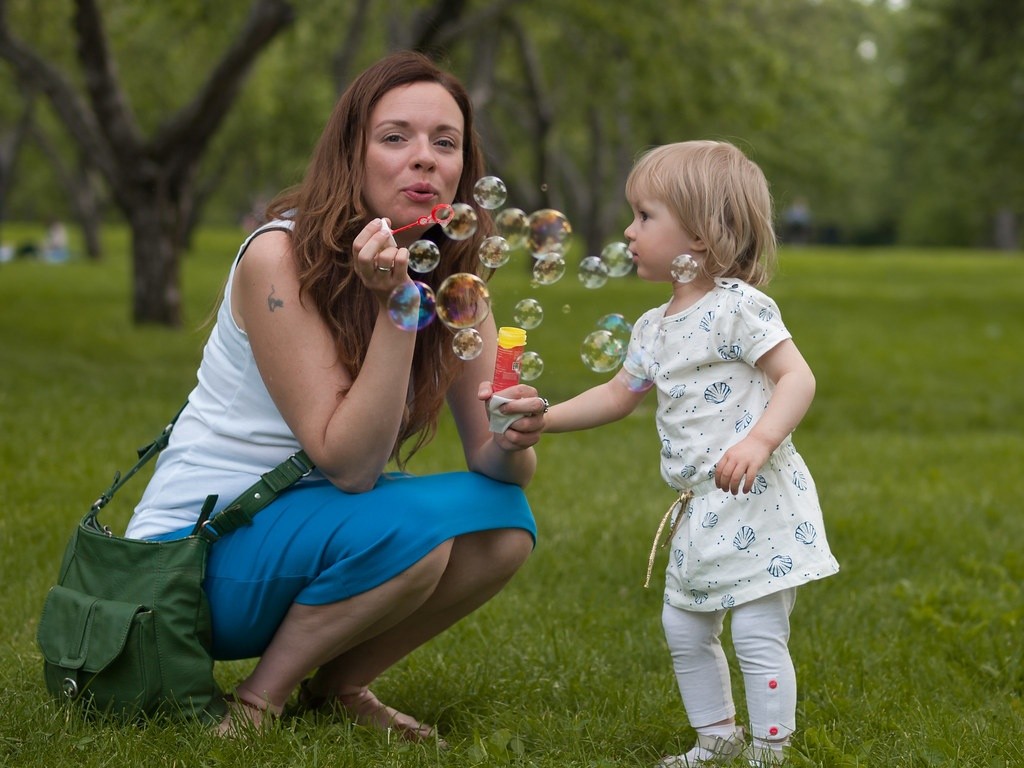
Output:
[122,54,549,747]
[535,140,840,768]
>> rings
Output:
[376,267,389,272]
[535,398,548,416]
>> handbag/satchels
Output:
[35,498,228,726]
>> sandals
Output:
[297,678,429,741]
[218,685,285,742]
[655,726,790,768]
